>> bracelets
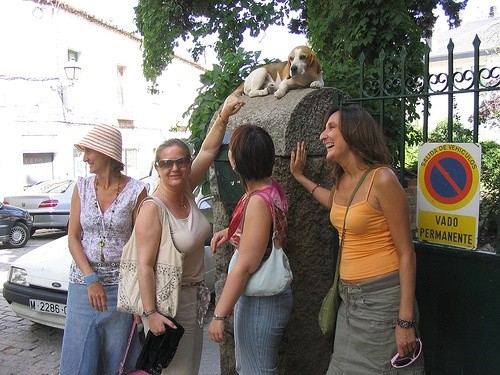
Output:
[217,109,231,125]
[311,184,321,195]
[83,273,98,285]
[142,309,156,317]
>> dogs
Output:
[229,45,325,99]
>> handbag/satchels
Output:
[227,190,293,297]
[134,316,184,375]
[116,197,183,316]
[318,287,341,333]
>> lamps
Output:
[56,57,82,94]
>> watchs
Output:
[395,317,416,329]
[212,313,226,320]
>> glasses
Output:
[391,339,422,368]
[156,157,190,169]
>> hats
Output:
[75,124,125,166]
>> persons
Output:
[203,123,295,375]
[290,104,427,375]
[134,95,245,375]
[57,124,154,375]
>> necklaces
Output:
[157,189,189,209]
[94,170,122,263]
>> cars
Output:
[2,175,218,332]
[3,178,76,235]
[0,201,36,249]
